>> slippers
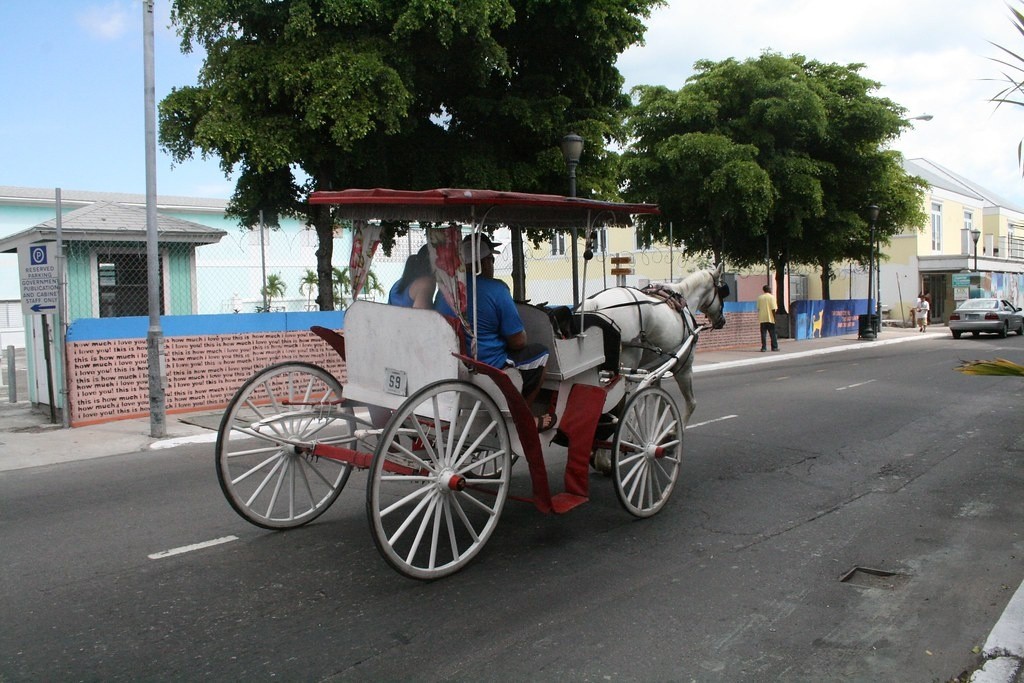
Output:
[533,411,558,432]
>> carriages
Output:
[213,186,730,582]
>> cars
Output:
[949,297,1024,340]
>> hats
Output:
[462,233,502,264]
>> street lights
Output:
[860,204,881,340]
[557,131,586,311]
[971,228,981,271]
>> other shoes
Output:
[762,348,766,351]
[920,328,922,332]
[923,330,926,332]
[772,348,780,351]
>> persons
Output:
[385,230,557,434]
[917,295,930,333]
[757,285,781,352]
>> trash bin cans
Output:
[857,314,879,338]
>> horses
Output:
[562,257,727,470]
